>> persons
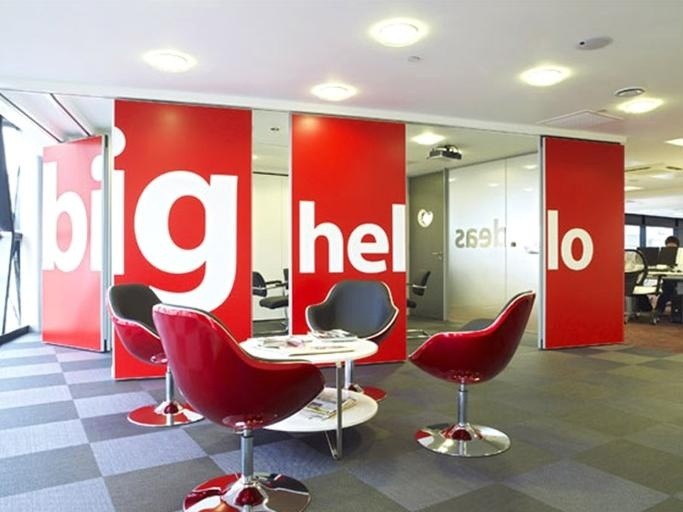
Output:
[652,235,682,324]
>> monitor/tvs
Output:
[636,247,659,265]
[659,246,678,265]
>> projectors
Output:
[428,147,463,161]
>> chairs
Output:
[408,291,535,458]
[624,248,667,324]
[281,268,289,326]
[152,302,324,511]
[406,270,432,339]
[305,279,399,404]
[252,272,289,334]
[105,283,204,428]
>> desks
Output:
[638,271,683,280]
